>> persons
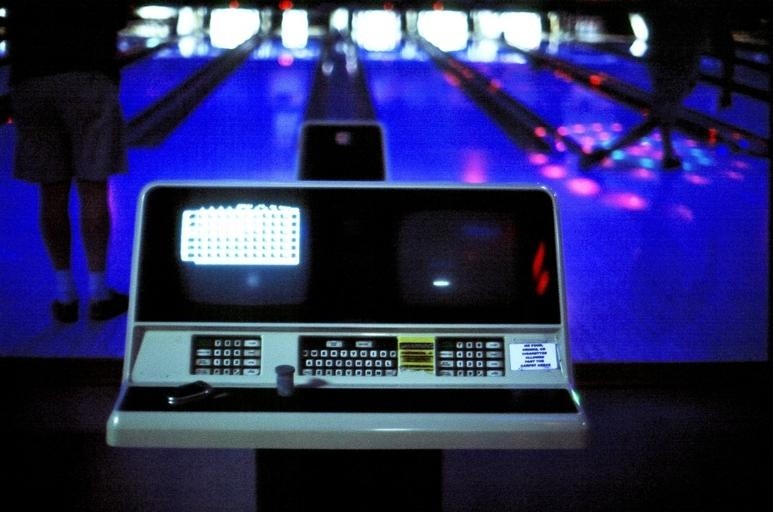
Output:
[3,0,139,323]
[576,1,737,176]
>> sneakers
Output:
[52,298,80,323]
[89,289,128,321]
